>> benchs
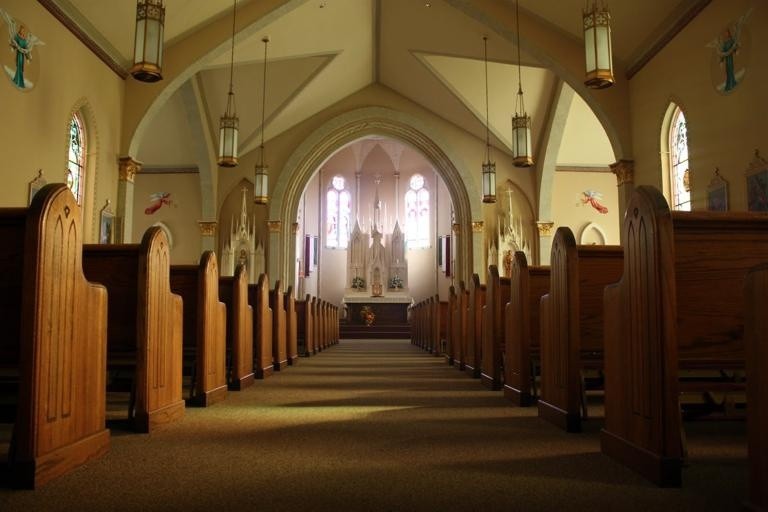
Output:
[82,224,185,433]
[408,250,551,408]
[537,226,623,432]
[170,250,338,410]
[0,184,111,490]
[595,185,768,510]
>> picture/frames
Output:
[705,168,730,211]
[27,168,48,206]
[743,149,768,210]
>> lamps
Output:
[130,0,270,205]
[481,1,615,204]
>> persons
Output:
[10,25,30,88]
[145,193,171,214]
[720,30,740,91]
[582,192,608,214]
